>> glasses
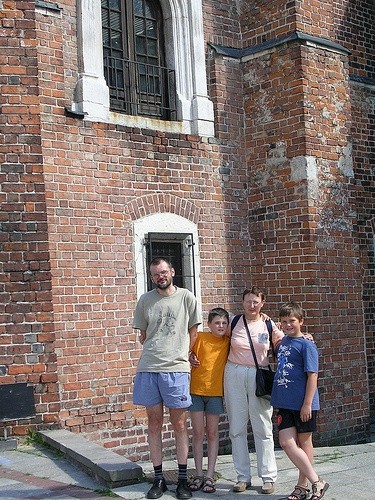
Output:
[150,268,171,279]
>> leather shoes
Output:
[176,483,192,499]
[147,477,167,499]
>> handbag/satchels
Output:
[255,369,275,400]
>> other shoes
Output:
[261,482,274,494]
[234,481,251,492]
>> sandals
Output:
[288,484,310,500]
[309,476,329,500]
[188,476,204,490]
[203,477,215,492]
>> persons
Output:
[269,301,329,500]
[189,286,313,494]
[132,257,202,499]
[188,308,230,493]
